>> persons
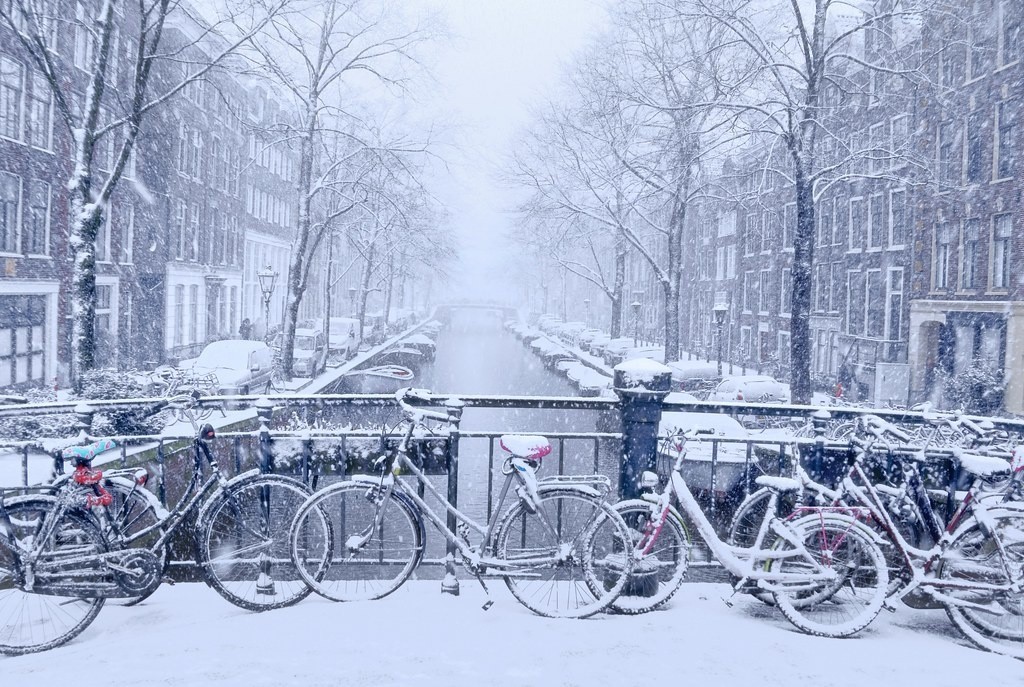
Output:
[925,356,1004,417]
[837,353,857,403]
[238,318,253,340]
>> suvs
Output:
[269,328,328,380]
[314,307,408,354]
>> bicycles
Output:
[270,344,286,393]
[0,395,334,656]
[727,401,1023,661]
[741,392,792,435]
[288,386,634,620]
[141,361,228,426]
[0,395,175,607]
[582,425,891,638]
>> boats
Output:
[415,320,443,339]
[344,365,414,394]
[371,348,422,376]
[504,320,614,396]
[394,333,436,362]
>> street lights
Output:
[711,303,729,381]
[257,266,280,345]
[584,297,591,328]
[347,285,357,317]
[631,297,642,348]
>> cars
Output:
[707,376,792,425]
[666,360,716,394]
[193,340,269,411]
[537,314,666,369]
